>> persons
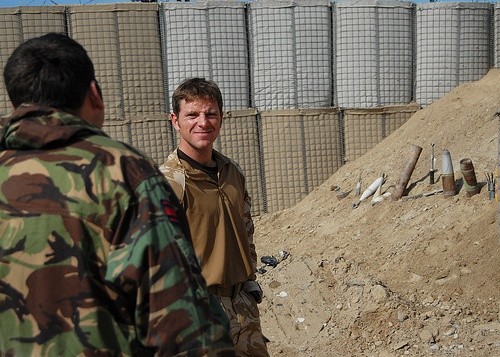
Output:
[157,78,272,357]
[0,31,237,357]
